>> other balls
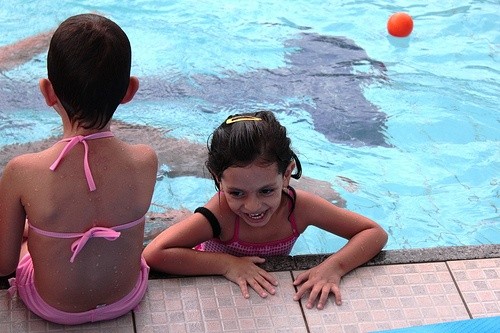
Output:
[388,12,413,37]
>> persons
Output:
[141,111,388,311]
[0,12,160,326]
